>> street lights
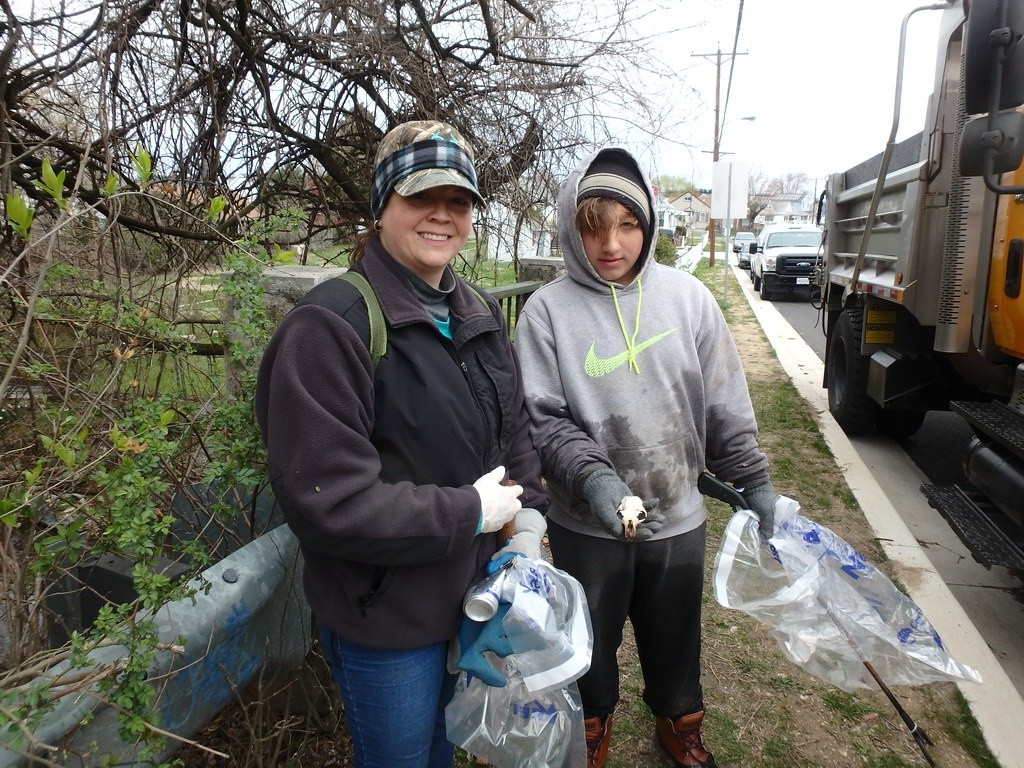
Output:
[724,116,755,305]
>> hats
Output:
[368,119,488,222]
[576,159,652,235]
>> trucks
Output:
[816,0,1024,573]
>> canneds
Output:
[461,567,506,622]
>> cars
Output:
[732,232,756,269]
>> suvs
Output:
[749,219,824,300]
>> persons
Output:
[257,122,552,768]
[515,146,775,768]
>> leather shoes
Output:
[656,710,719,768]
[584,714,613,768]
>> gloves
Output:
[486,508,547,575]
[472,465,523,537]
[582,468,666,542]
[732,480,780,540]
